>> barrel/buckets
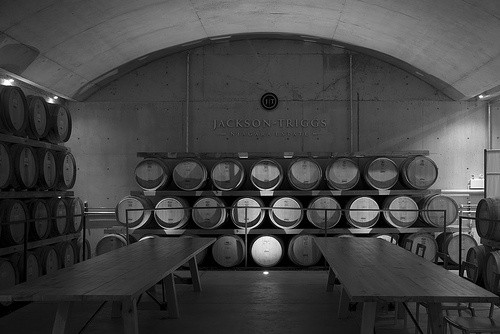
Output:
[95,154,500,297]
[0,84,91,306]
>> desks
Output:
[0,237,216,334]
[312,237,499,334]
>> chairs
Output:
[390,235,500,334]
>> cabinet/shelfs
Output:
[104,151,471,272]
[0,132,80,318]
